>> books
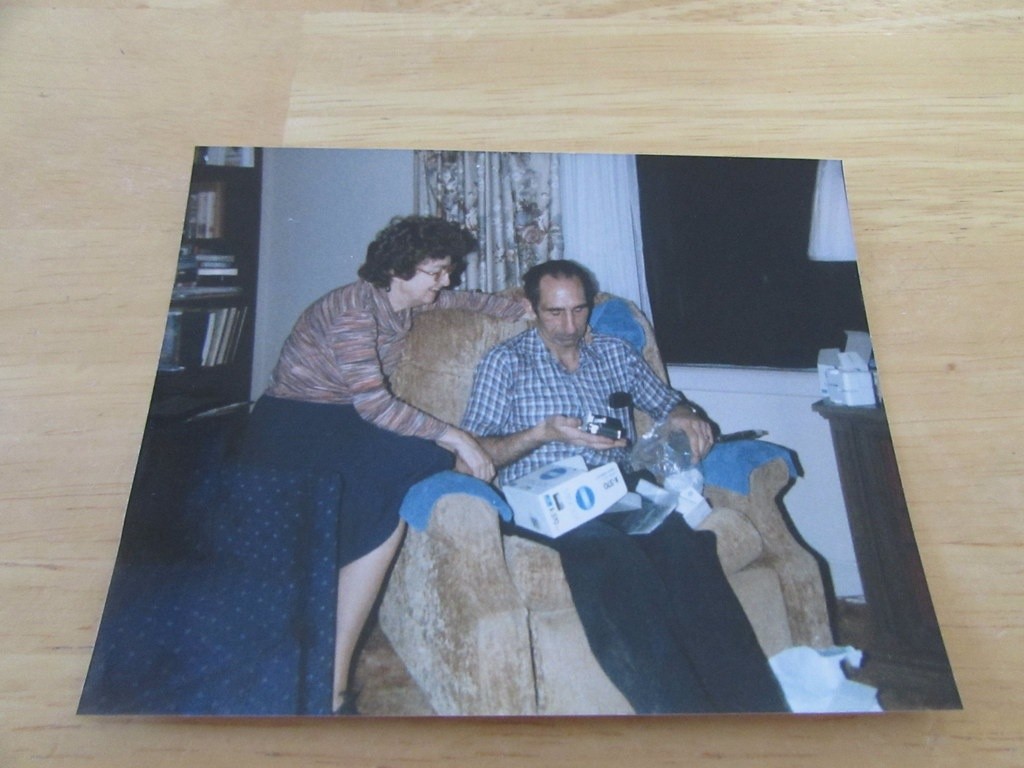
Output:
[190,147,257,170]
[158,306,251,371]
[183,180,227,239]
[169,244,242,300]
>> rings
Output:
[705,435,709,440]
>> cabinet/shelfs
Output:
[815,394,967,711]
[156,148,268,394]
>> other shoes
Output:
[330,689,363,715]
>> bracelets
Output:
[681,402,699,414]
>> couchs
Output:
[379,288,834,718]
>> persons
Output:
[453,260,792,715]
[246,212,496,715]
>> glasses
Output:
[414,258,459,283]
